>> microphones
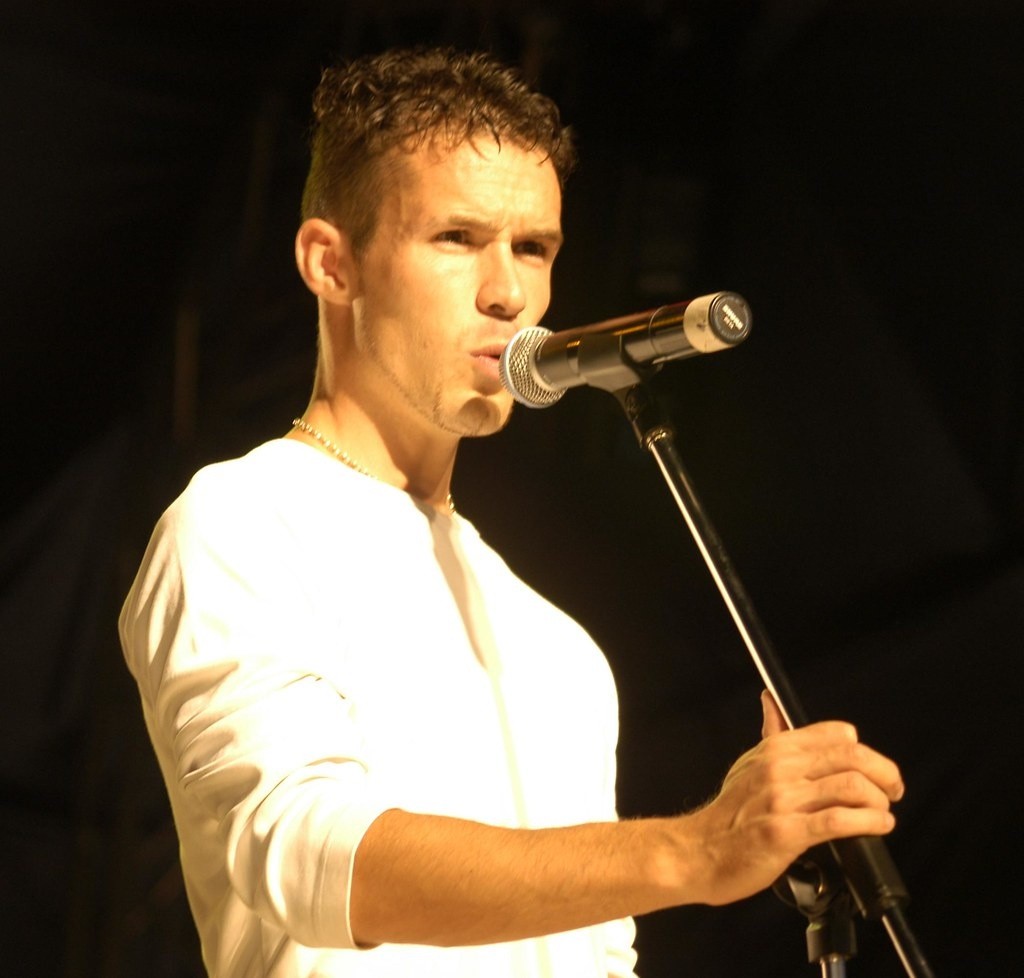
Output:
[499,290,751,408]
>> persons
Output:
[117,44,905,978]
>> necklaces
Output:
[292,416,456,513]
